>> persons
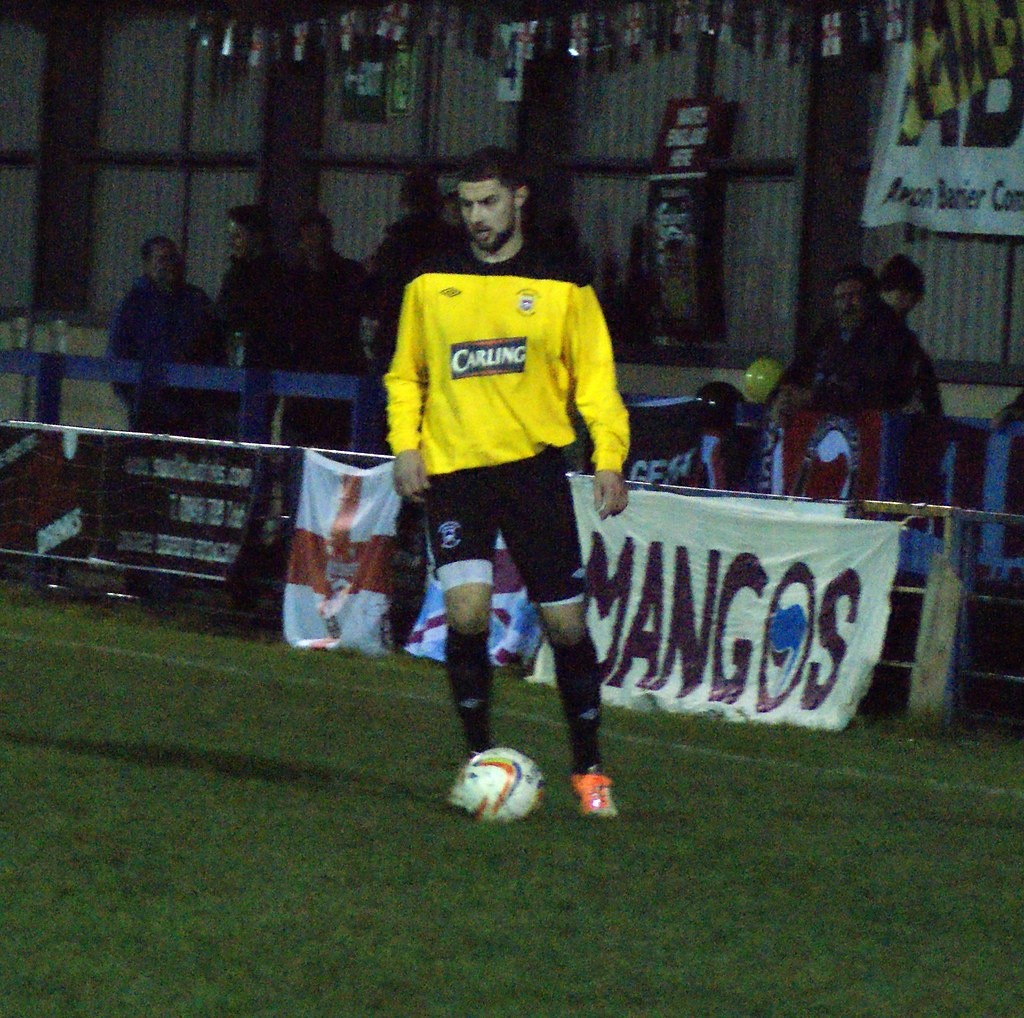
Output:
[217,205,282,425]
[764,256,944,409]
[365,147,631,818]
[104,237,229,436]
[259,212,362,452]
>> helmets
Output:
[693,380,742,427]
[746,356,783,401]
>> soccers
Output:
[461,747,547,825]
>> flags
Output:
[192,0,907,58]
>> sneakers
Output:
[569,770,619,819]
